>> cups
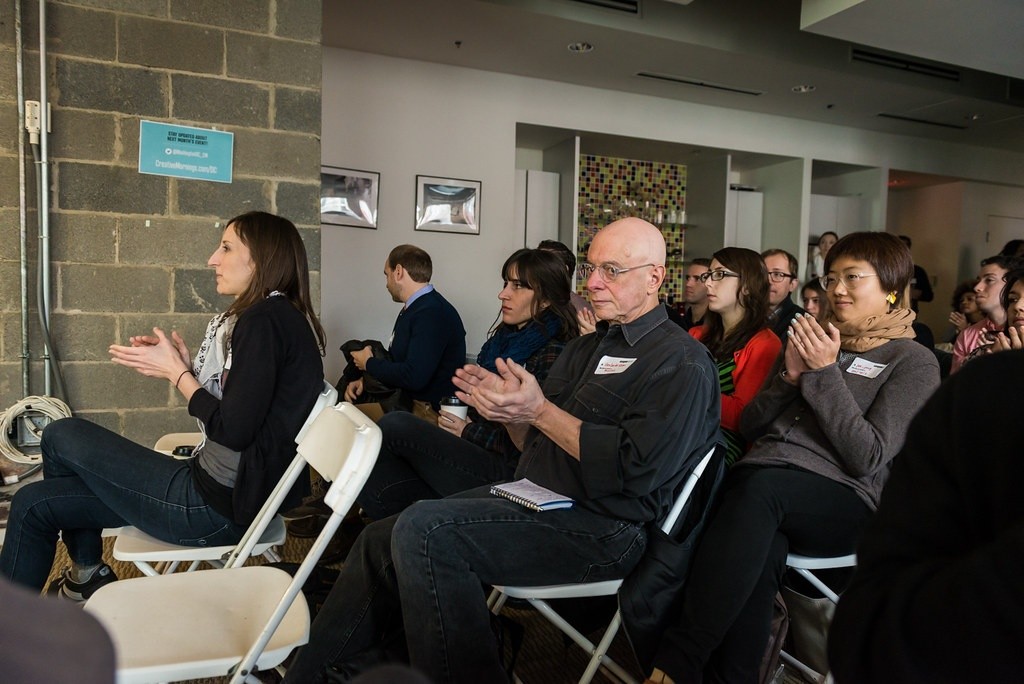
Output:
[172,445,196,460]
[439,395,469,423]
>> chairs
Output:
[80,364,856,684]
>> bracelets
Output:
[175,370,191,388]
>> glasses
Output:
[701,270,738,282]
[579,263,655,282]
[768,271,792,282]
[819,272,879,291]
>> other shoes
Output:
[317,527,362,565]
[287,515,328,537]
[283,495,333,517]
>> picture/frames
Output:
[413,173,482,235]
[320,163,381,229]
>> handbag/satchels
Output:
[325,648,392,684]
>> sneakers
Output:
[49,564,119,607]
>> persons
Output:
[285,245,465,537]
[567,230,1024,684]
[280,218,720,683]
[319,249,580,568]
[1,212,324,605]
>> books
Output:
[490,478,576,513]
